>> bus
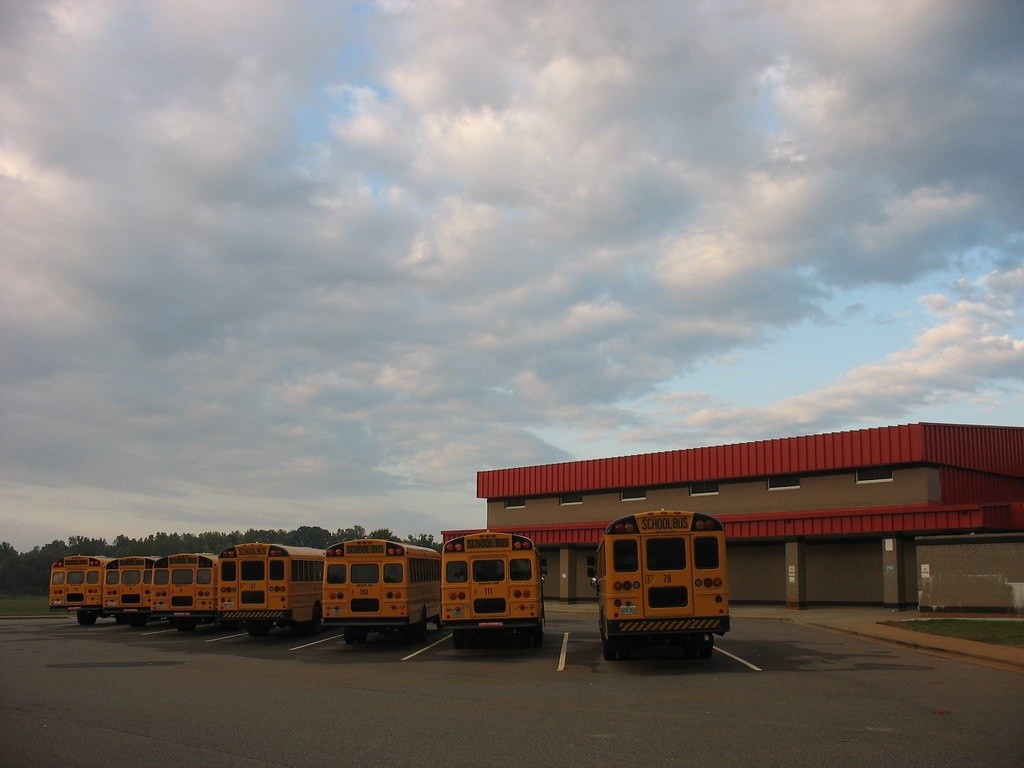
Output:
[591,506,732,662]
[101,554,161,626]
[215,541,325,638]
[150,552,220,632]
[319,539,441,644]
[49,555,117,626]
[437,530,548,650]
